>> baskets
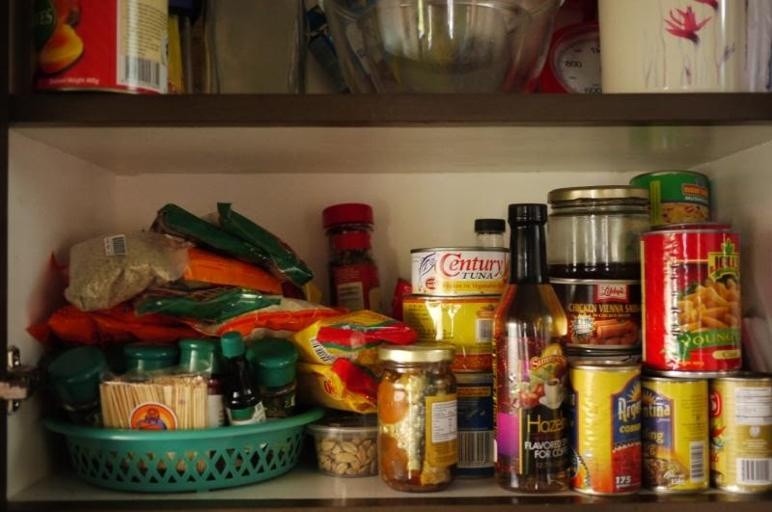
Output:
[35,408,328,496]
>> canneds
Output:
[376,247,512,492]
[548,170,772,495]
[25,0,170,98]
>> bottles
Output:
[547,181,654,357]
[320,203,382,313]
[494,197,569,492]
[49,333,302,421]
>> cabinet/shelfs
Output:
[1,93,772,512]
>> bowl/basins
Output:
[315,0,567,94]
